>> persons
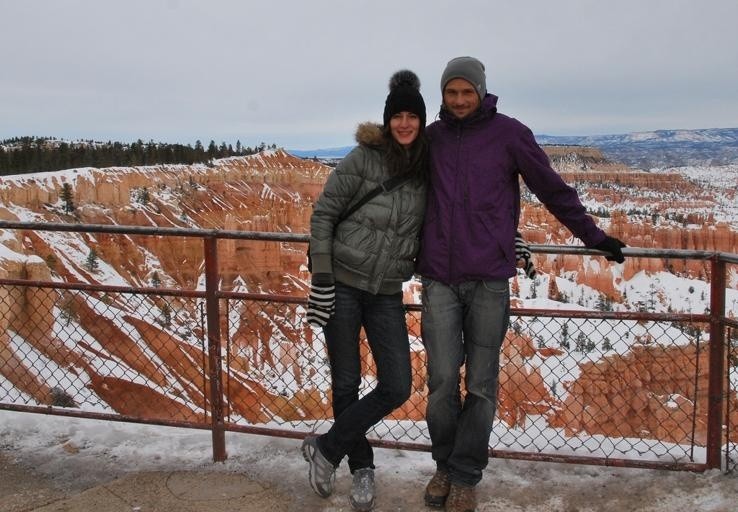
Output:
[307,57,627,512]
[301,70,537,512]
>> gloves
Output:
[593,235,627,263]
[513,231,537,280]
[306,273,336,328]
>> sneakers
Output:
[445,481,477,511]
[424,470,450,507]
[348,466,375,511]
[300,435,336,499]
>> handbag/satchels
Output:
[306,226,335,271]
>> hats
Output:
[441,57,486,103]
[383,70,426,136]
